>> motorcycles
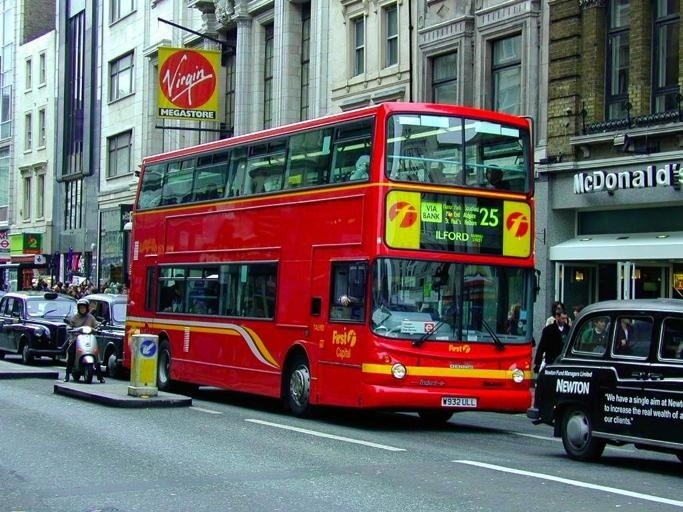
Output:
[61,316,111,384]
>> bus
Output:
[118,97,541,428]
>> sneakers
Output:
[99,378,106,384]
[63,374,70,383]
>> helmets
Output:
[77,298,91,315]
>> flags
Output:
[155,45,223,123]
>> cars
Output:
[0,290,78,365]
[525,295,682,468]
[64,294,128,376]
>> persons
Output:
[499,300,637,376]
[332,264,369,323]
[481,163,512,191]
[158,266,274,316]
[36,278,126,299]
[349,155,372,181]
[63,299,108,384]
